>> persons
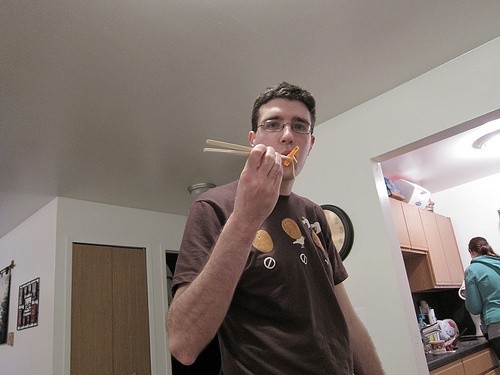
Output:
[463,236,500,360]
[165,82,383,375]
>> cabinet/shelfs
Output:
[429,347,500,375]
[389,197,428,260]
[404,208,465,293]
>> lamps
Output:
[187,183,217,197]
[473,129,500,148]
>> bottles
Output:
[418,318,427,331]
[429,340,447,355]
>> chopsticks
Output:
[203,139,294,160]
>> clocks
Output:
[319,204,354,260]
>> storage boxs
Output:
[384,177,405,201]
[394,179,430,208]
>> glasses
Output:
[254,119,312,134]
